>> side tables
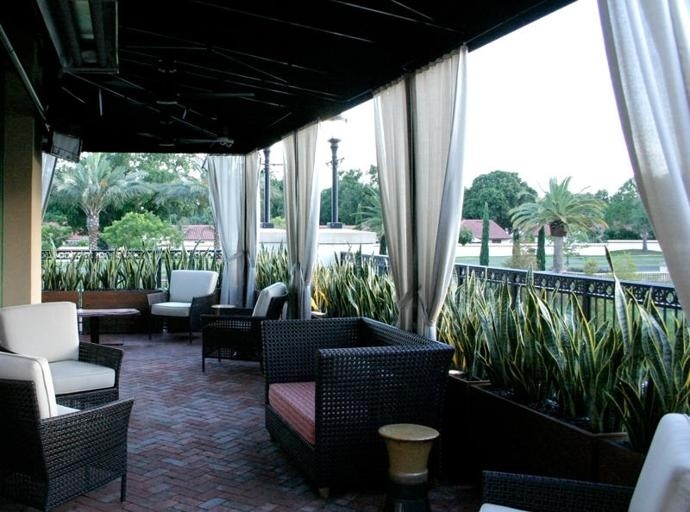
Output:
[211,304,236,323]
[377,423,440,511]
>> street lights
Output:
[327,138,343,228]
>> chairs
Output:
[200,282,289,376]
[261,317,455,502]
[479,411,690,512]
[0,300,122,410]
[145,270,220,344]
[0,352,134,512]
